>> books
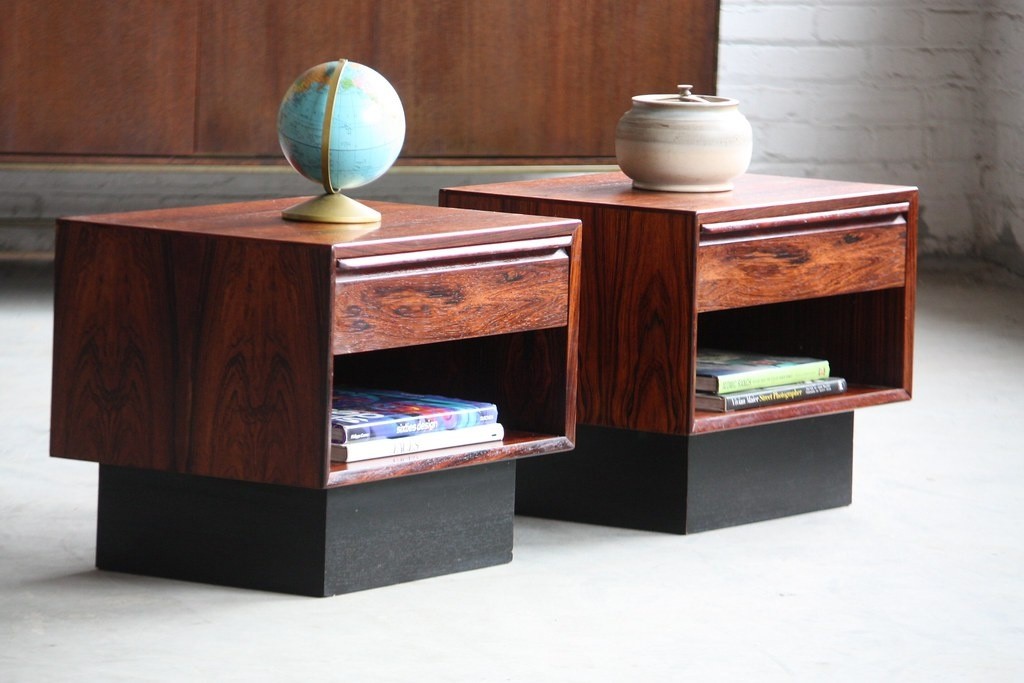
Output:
[331,387,504,464]
[696,349,848,414]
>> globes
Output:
[275,58,406,225]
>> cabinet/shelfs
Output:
[439,164,920,439]
[46,198,585,491]
